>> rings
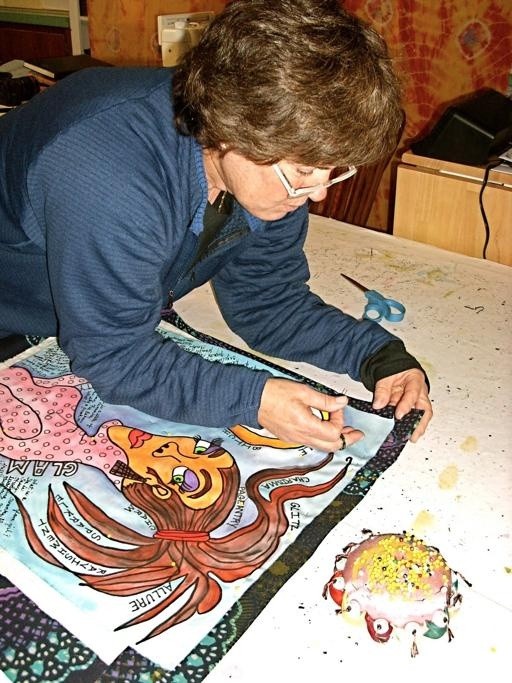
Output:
[339,433,346,451]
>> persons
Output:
[0,0,433,453]
[0,366,353,645]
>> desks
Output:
[1,211,512,682]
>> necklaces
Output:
[218,190,228,213]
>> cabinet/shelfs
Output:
[391,149,512,264]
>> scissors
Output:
[341,273,406,323]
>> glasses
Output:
[274,163,357,200]
